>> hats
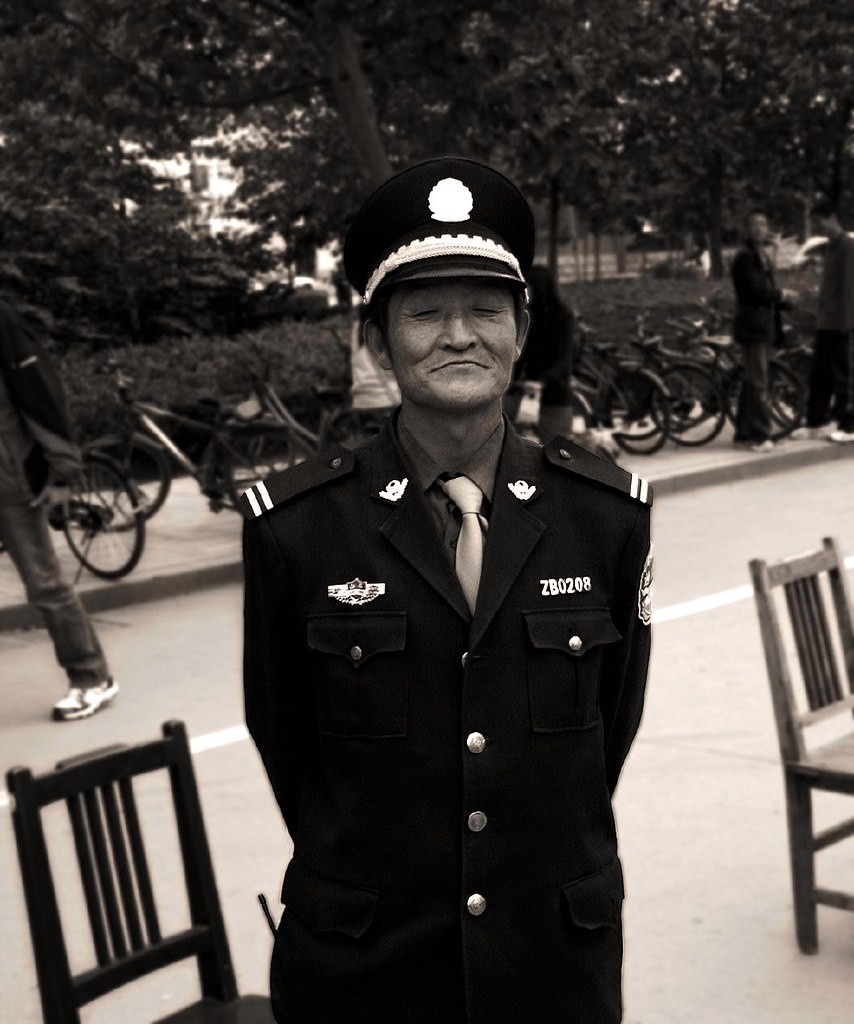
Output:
[343,156,535,313]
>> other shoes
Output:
[732,433,748,448]
[788,426,815,440]
[815,419,854,441]
[748,433,774,453]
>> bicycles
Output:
[186,282,854,499]
[61,344,317,533]
[48,442,146,588]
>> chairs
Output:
[7,718,277,1024]
[750,536,854,953]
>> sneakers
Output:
[51,675,121,721]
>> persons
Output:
[351,298,401,434]
[792,197,854,441]
[513,264,575,446]
[237,154,654,1023]
[729,208,799,453]
[0,298,118,725]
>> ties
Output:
[435,475,488,616]
